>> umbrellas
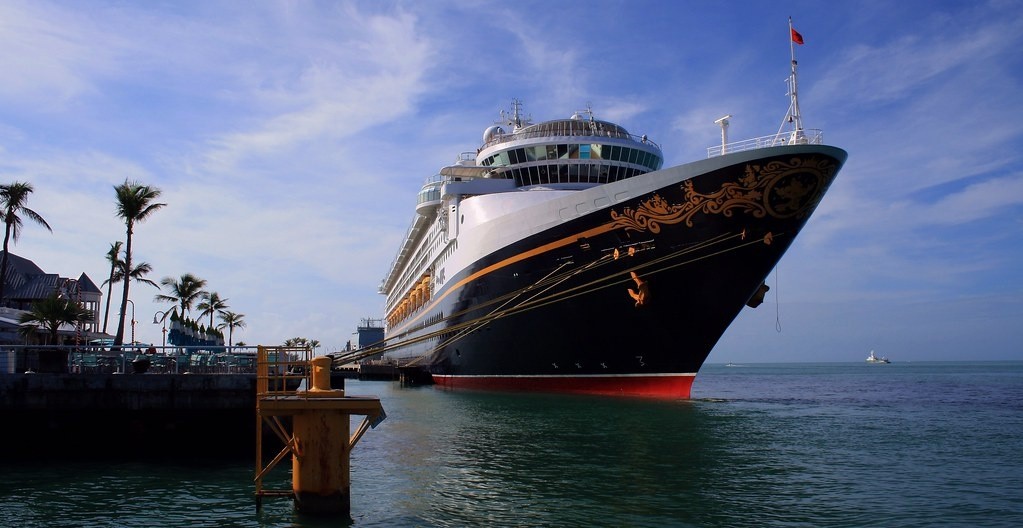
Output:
[167,308,226,354]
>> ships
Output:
[376,18,849,399]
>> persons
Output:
[148,343,156,355]
[137,348,142,354]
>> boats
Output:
[865,350,888,363]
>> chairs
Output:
[72,353,279,374]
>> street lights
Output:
[118,299,135,351]
[151,310,166,355]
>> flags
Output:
[792,28,804,45]
